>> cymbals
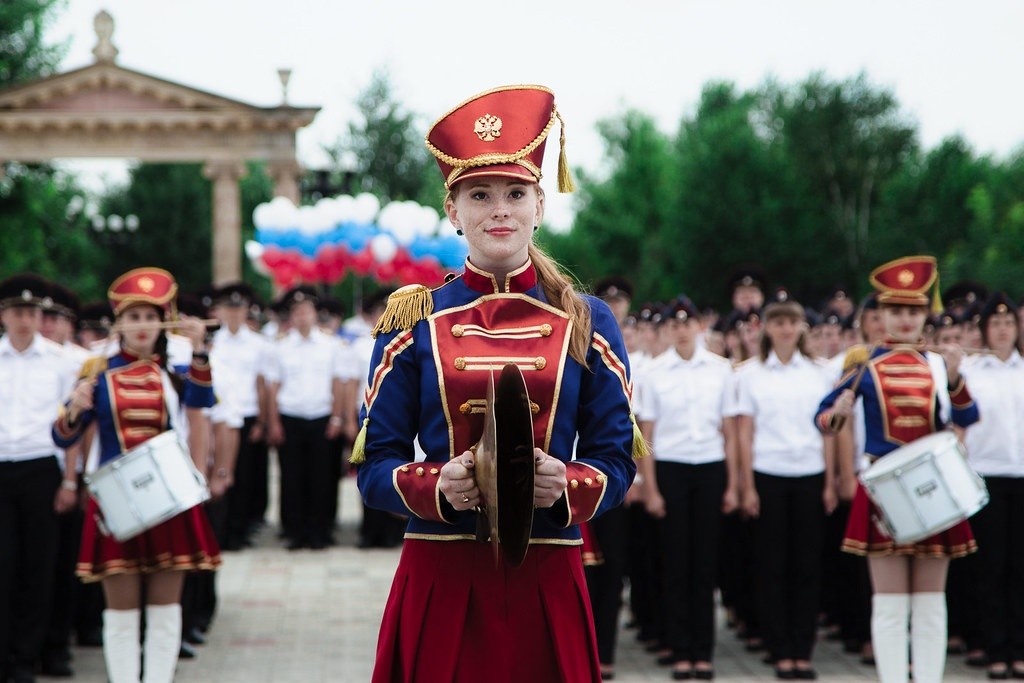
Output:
[504,363,537,568]
[475,365,502,573]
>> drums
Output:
[85,429,212,543]
[854,429,990,546]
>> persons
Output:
[354,84,649,683]
[0,268,1024,683]
[51,267,219,683]
[814,255,981,683]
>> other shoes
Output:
[0,524,410,683]
[596,580,1024,683]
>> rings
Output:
[462,493,469,504]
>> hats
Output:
[107,266,179,334]
[595,265,1024,354]
[0,272,395,343]
[870,254,945,317]
[424,85,576,193]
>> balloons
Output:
[246,192,471,290]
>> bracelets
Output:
[255,419,264,427]
[217,468,225,476]
[330,416,343,426]
[60,480,77,491]
[634,475,643,483]
